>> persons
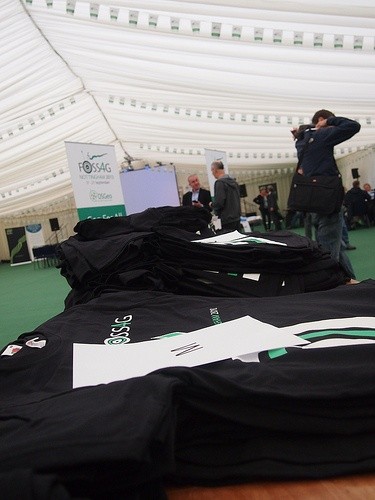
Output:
[344,180,375,224]
[253,186,273,233]
[304,208,357,250]
[265,185,282,231]
[209,161,241,229]
[296,109,361,262]
[183,175,213,212]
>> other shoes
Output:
[346,244,356,250]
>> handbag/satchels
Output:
[287,173,343,214]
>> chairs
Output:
[32,243,61,269]
